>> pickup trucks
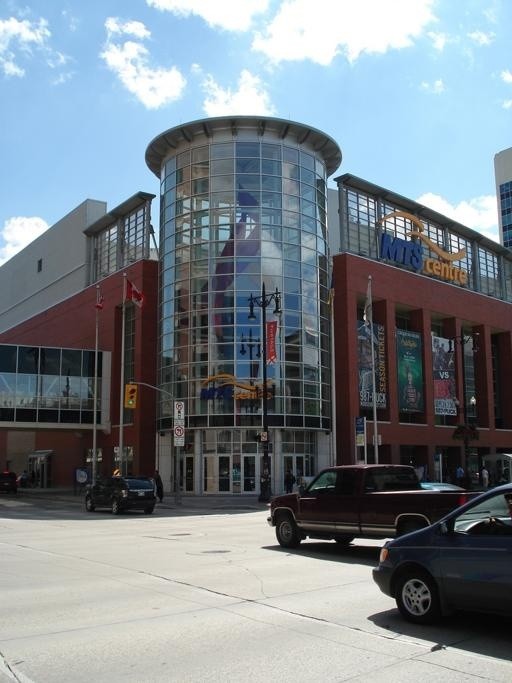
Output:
[268,464,487,547]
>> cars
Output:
[0,472,17,494]
[373,483,512,625]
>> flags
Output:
[125,279,145,309]
[89,296,105,312]
[364,280,373,328]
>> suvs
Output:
[86,475,157,513]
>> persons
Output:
[283,469,295,494]
[482,467,489,487]
[456,463,465,488]
[403,372,420,407]
[497,494,512,536]
[150,470,164,503]
[16,470,30,488]
[148,474,157,499]
[449,464,458,484]
[297,474,307,493]
[479,465,484,487]
[258,462,271,503]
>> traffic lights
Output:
[125,385,137,408]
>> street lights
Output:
[453,397,477,488]
[248,284,282,502]
[240,328,261,426]
